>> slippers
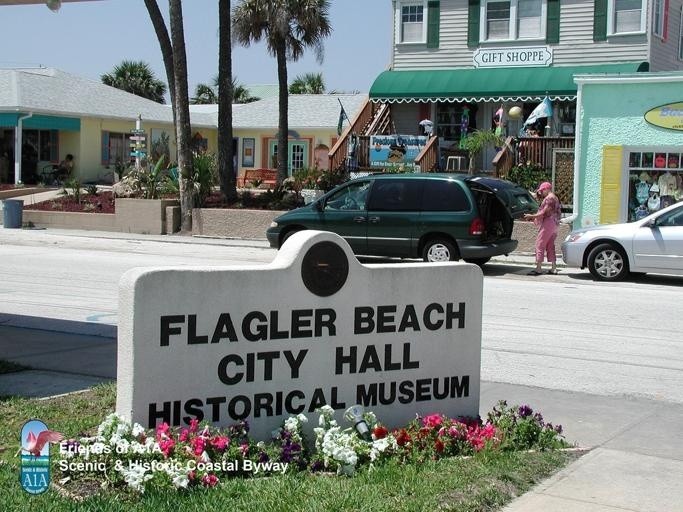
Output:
[527,271,542,275]
[546,270,557,275]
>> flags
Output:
[336,107,347,136]
[522,98,553,127]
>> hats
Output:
[538,182,552,192]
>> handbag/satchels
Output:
[648,197,660,210]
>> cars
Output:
[559,201,683,282]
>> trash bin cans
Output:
[3,200,23,228]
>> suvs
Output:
[262,174,537,269]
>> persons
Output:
[48,152,74,185]
[523,181,562,276]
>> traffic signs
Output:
[127,129,146,159]
